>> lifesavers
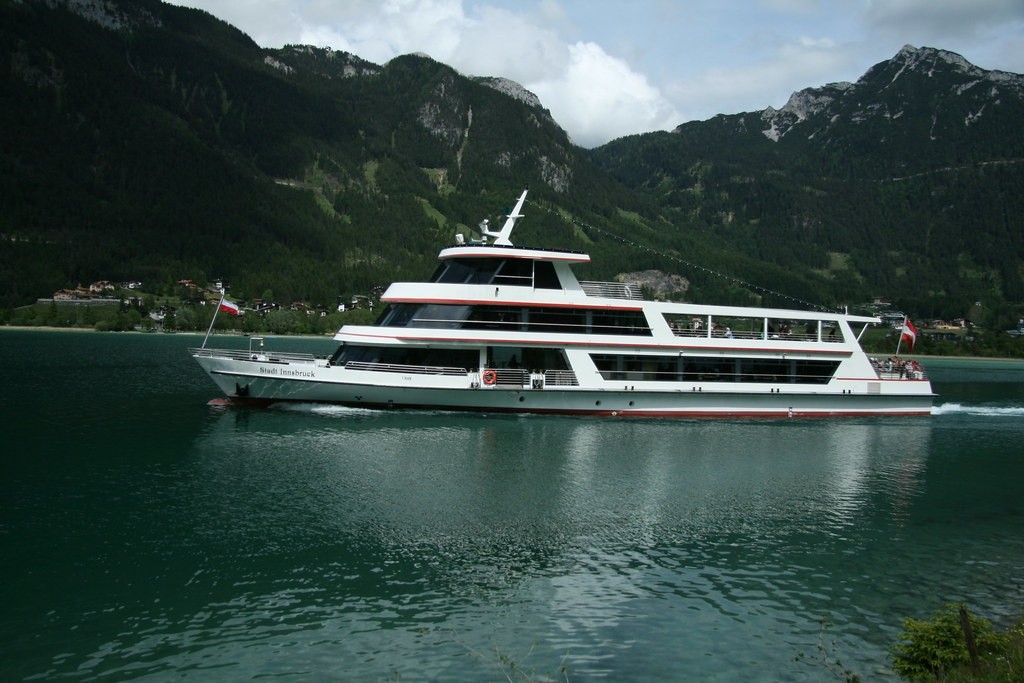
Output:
[483,371,496,384]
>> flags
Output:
[219,299,239,314]
[901,315,918,352]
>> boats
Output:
[185,189,941,418]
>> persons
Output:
[507,354,523,369]
[668,315,837,341]
[870,356,924,380]
